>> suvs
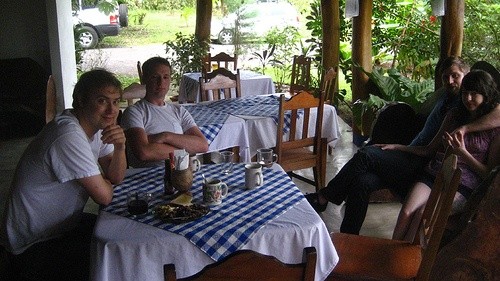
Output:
[71,0,128,50]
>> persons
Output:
[371,69,500,240]
[0,68,128,281]
[304,58,471,235]
[119,56,209,169]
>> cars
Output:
[210,0,301,45]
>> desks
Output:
[89,161,340,281]
[178,103,249,162]
[180,69,274,104]
[199,92,342,190]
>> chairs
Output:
[199,52,242,101]
[164,246,317,281]
[271,54,338,192]
[323,146,463,281]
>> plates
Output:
[159,205,209,223]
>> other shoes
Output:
[304,193,328,213]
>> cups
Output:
[127,188,149,216]
[218,150,234,174]
[256,148,278,167]
[187,154,201,172]
[245,163,263,189]
[202,178,228,206]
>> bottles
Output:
[163,159,174,193]
